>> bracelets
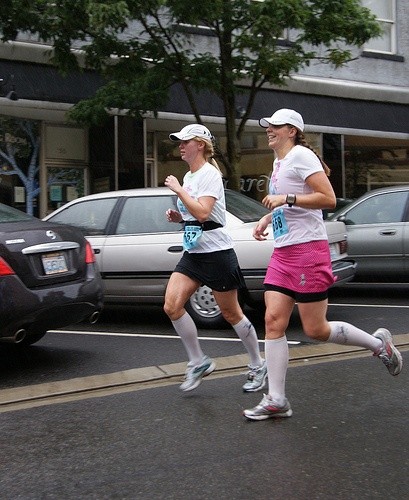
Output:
[286,193,296,208]
[179,219,185,224]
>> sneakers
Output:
[372,328,403,376]
[243,360,267,392]
[180,355,217,391]
[242,392,293,420]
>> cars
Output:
[0,202,103,358]
[41,186,358,330]
[327,184,409,284]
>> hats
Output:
[169,124,212,142]
[259,108,304,133]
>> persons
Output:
[162,122,269,393]
[243,108,402,421]
